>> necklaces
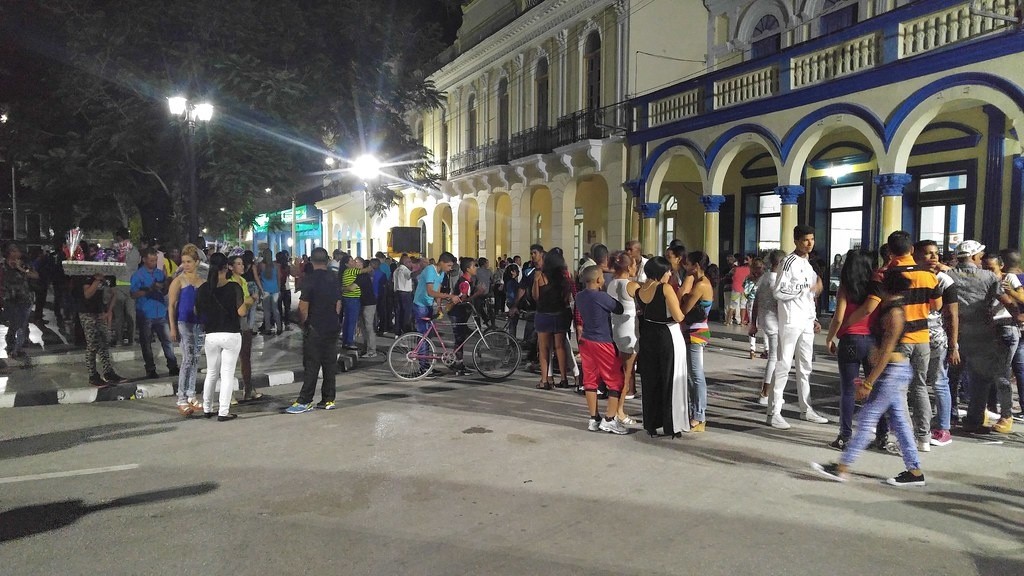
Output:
[641,280,655,292]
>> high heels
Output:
[646,428,658,438]
[689,420,706,433]
[671,432,682,439]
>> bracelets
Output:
[852,376,873,391]
[948,343,960,352]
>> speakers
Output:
[391,227,420,253]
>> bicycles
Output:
[388,301,522,381]
[500,308,570,374]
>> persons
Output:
[167,243,203,416]
[193,251,259,423]
[826,230,1024,456]
[765,225,828,430]
[808,269,929,488]
[284,246,344,415]
[0,226,574,405]
[574,238,787,440]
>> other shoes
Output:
[625,391,640,399]
[204,411,217,418]
[360,351,377,358]
[619,415,642,425]
[342,344,362,350]
[178,397,203,416]
[993,417,1013,432]
[536,381,550,390]
[453,364,477,376]
[556,380,569,388]
[417,366,443,379]
[231,393,238,405]
[88,370,127,387]
[243,387,263,400]
[218,413,237,421]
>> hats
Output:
[956,239,987,257]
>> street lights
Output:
[265,188,297,265]
[324,156,379,261]
[166,95,213,245]
[220,207,242,248]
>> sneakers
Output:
[599,415,629,435]
[766,414,790,430]
[867,435,887,453]
[588,419,601,432]
[885,441,904,456]
[286,403,314,414]
[886,471,926,486]
[827,436,850,451]
[811,462,847,482]
[799,408,828,423]
[317,400,336,410]
[917,441,931,452]
[758,393,786,405]
[930,428,953,446]
